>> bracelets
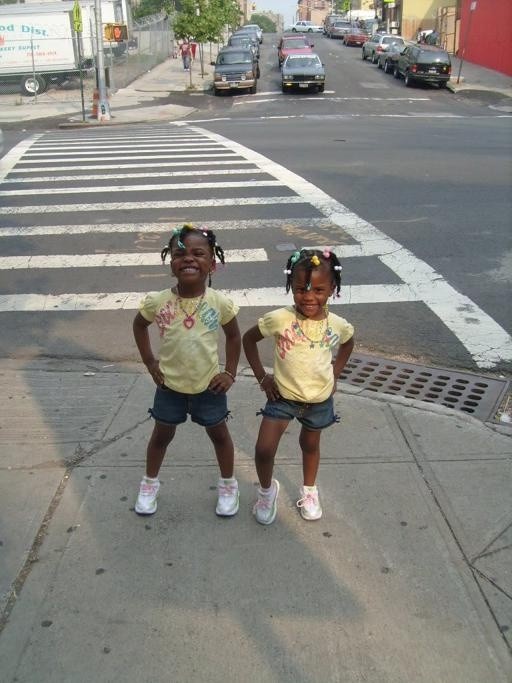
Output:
[224,369,236,383]
[259,371,268,385]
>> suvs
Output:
[362,34,451,88]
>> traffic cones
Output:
[88,87,99,119]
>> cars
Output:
[290,16,368,46]
[210,23,263,95]
[278,33,325,92]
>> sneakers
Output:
[299,485,322,521]
[255,479,280,525]
[215,476,240,517]
[134,476,159,516]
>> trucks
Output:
[0,0,133,95]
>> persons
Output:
[131,226,241,519]
[419,30,437,45]
[244,249,354,525]
[179,39,192,71]
[353,19,365,29]
